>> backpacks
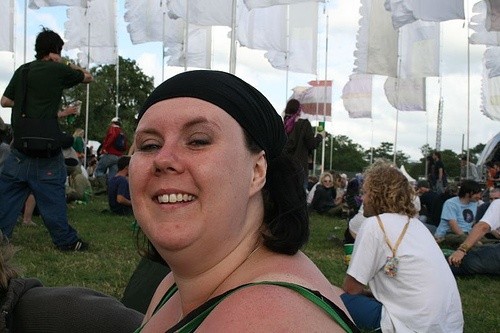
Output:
[116,130,126,151]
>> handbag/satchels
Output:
[14,115,62,158]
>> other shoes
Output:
[65,240,88,252]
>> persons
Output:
[0,106,171,333]
[282,98,500,279]
[0,28,93,251]
[130,70,360,333]
[333,160,463,333]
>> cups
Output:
[344,244,354,265]
[317,126,323,133]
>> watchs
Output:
[460,243,469,251]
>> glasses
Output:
[323,180,332,182]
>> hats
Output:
[110,117,122,125]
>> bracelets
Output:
[457,247,467,255]
